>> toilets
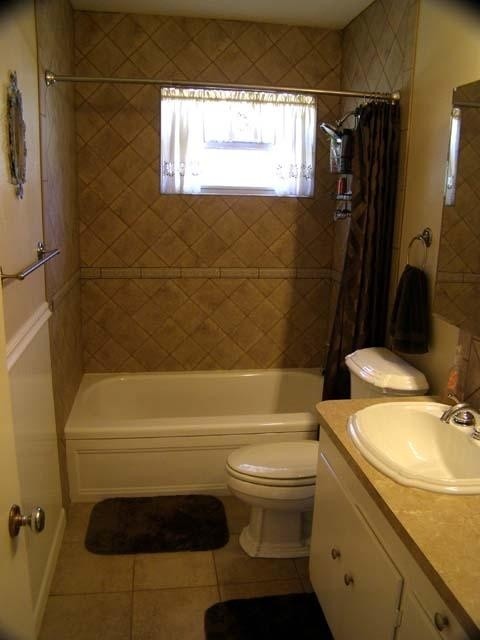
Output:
[220,348,430,560]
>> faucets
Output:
[437,403,480,438]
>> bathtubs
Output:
[66,368,326,501]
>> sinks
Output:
[346,400,479,498]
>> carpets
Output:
[204,593,334,640]
[85,495,229,555]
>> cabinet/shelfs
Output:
[309,425,470,640]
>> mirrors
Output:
[433,79,480,345]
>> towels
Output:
[390,264,430,355]
